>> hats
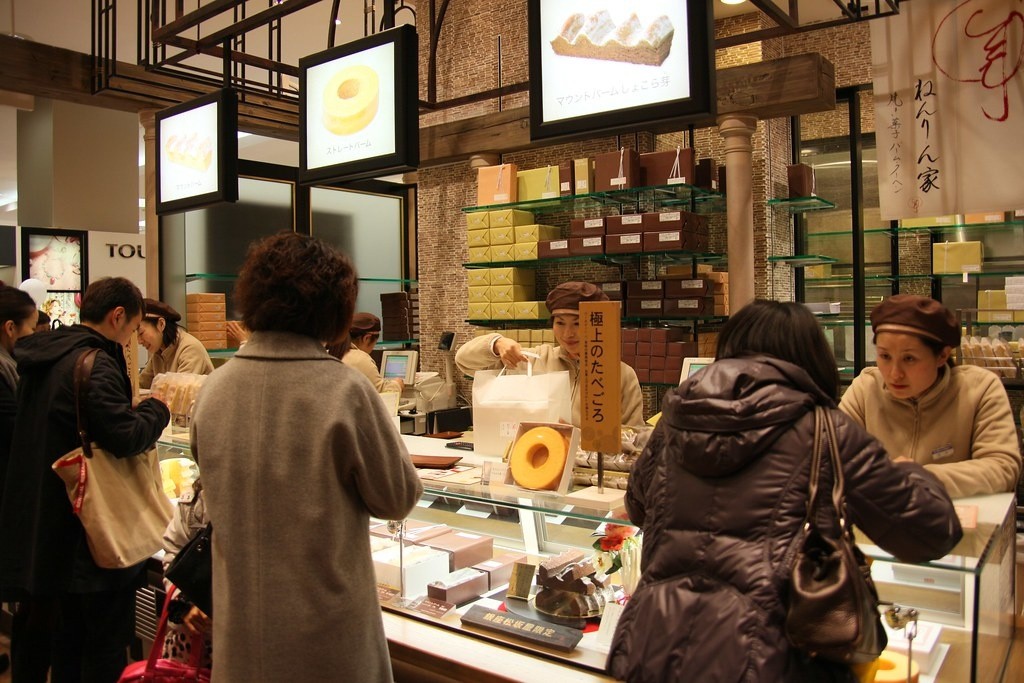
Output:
[545,281,610,316]
[347,312,382,335]
[868,294,964,350]
[142,298,181,323]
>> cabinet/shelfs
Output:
[768,195,841,328]
[154,423,1016,683]
[182,273,419,354]
[804,220,1023,392]
[461,183,729,389]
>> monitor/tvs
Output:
[679,358,716,386]
[380,351,418,386]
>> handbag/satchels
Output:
[770,407,887,664]
[471,351,572,461]
[164,521,212,621]
[118,585,215,683]
[50,348,173,567]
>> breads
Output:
[953,343,1016,379]
[166,135,212,170]
[872,650,921,683]
[510,426,569,490]
[549,8,674,67]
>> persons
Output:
[189,231,424,683]
[837,295,1022,497]
[605,299,964,683]
[454,280,647,428]
[0,274,404,683]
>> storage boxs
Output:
[933,241,984,274]
[186,293,246,350]
[804,264,831,279]
[977,276,1022,323]
[379,292,421,342]
[786,163,816,198]
[466,147,728,384]
[368,515,529,606]
[502,421,581,498]
[804,302,840,315]
[879,614,944,675]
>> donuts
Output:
[323,65,378,135]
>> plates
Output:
[529,597,605,618]
